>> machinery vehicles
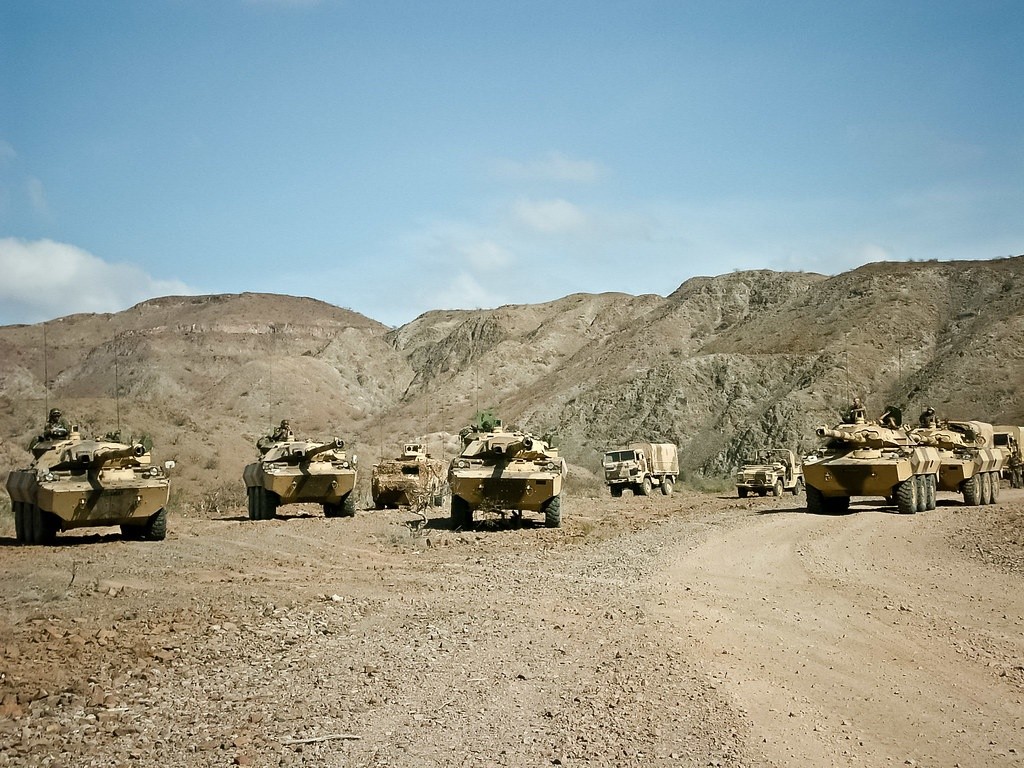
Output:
[445,418,568,530]
[371,443,446,507]
[6,425,175,546]
[243,435,358,521]
[802,405,1024,514]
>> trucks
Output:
[735,449,806,498]
[602,438,679,496]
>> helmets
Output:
[51,409,61,416]
[483,421,492,428]
[927,407,935,414]
[281,420,289,426]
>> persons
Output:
[879,409,897,428]
[272,420,293,442]
[44,408,68,440]
[920,407,941,428]
[1006,451,1024,488]
[850,397,867,421]
[482,421,492,431]
[760,451,788,466]
